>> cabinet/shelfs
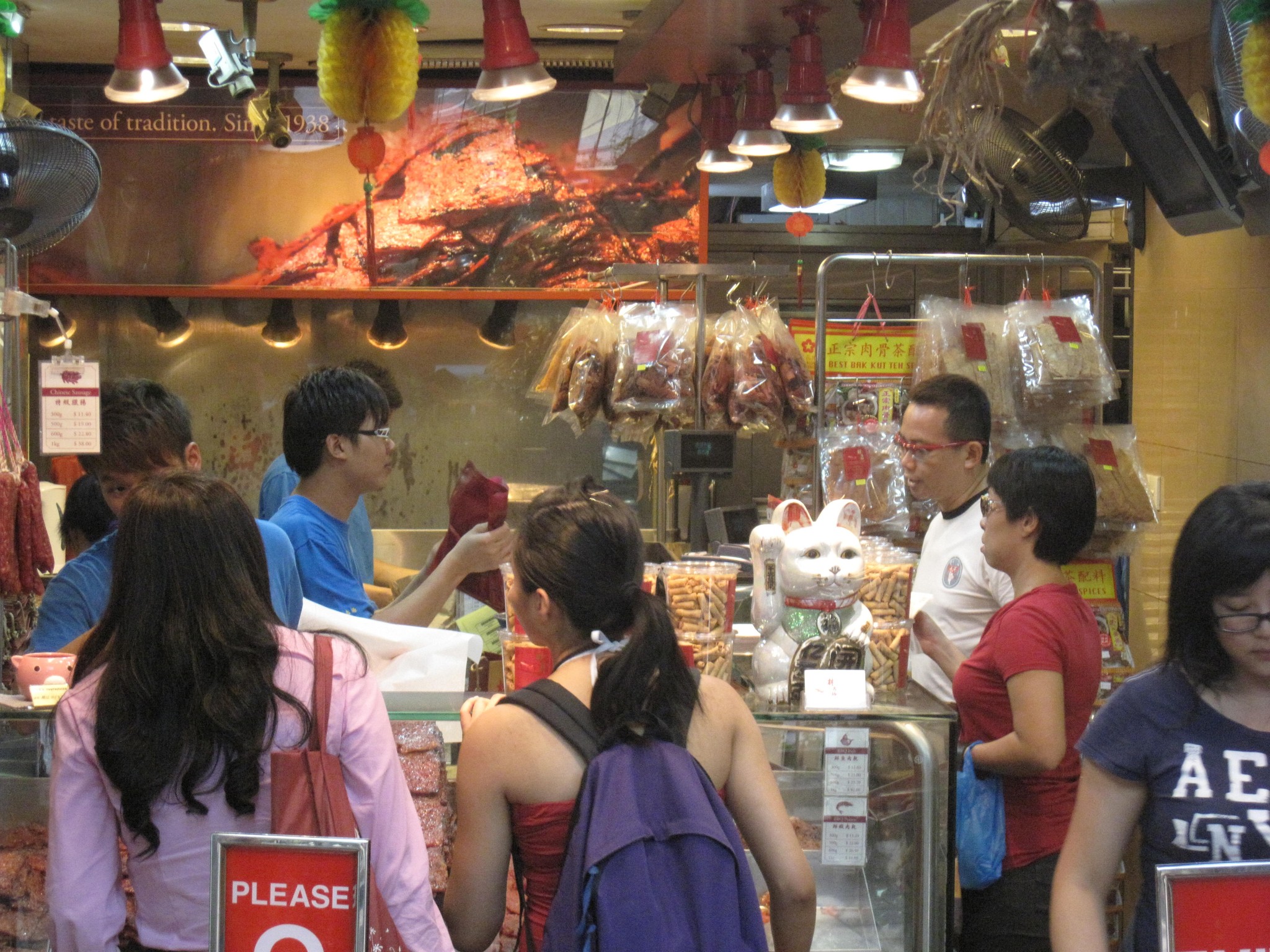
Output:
[0,678,959,950]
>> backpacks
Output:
[484,666,768,952]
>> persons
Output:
[912,446,1103,952]
[28,380,303,655]
[258,359,422,610]
[1049,482,1269,952]
[442,483,817,952]
[269,365,514,629]
[897,373,1013,709]
[60,474,121,557]
[43,470,457,952]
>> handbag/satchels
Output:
[958,739,1006,891]
[270,635,415,952]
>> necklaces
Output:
[552,636,617,672]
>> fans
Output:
[1209,0,1270,191]
[0,115,102,265]
[955,104,1146,249]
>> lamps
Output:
[473,1,557,103]
[695,74,753,174]
[36,295,77,348]
[260,297,302,349]
[768,3,843,133]
[105,1,190,105]
[726,42,792,156]
[143,296,196,349]
[759,145,905,216]
[840,0,927,105]
[367,300,408,349]
[476,300,520,349]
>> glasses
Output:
[1204,602,1267,635]
[893,432,986,457]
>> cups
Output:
[7,649,77,699]
